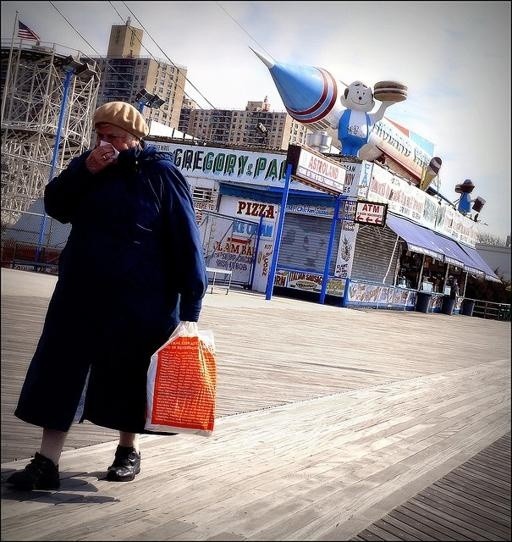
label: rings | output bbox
[102,154,107,161]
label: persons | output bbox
[0,99,210,491]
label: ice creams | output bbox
[422,157,442,191]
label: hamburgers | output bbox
[296,281,317,290]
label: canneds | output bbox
[273,273,287,287]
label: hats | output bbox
[93,101,149,140]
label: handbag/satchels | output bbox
[144,318,218,437]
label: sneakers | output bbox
[106,446,142,482]
[7,452,61,490]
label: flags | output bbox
[17,21,42,42]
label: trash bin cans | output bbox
[462,299,474,316]
[442,295,455,315]
[416,292,432,313]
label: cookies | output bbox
[374,81,408,102]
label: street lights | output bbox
[34,53,98,264]
